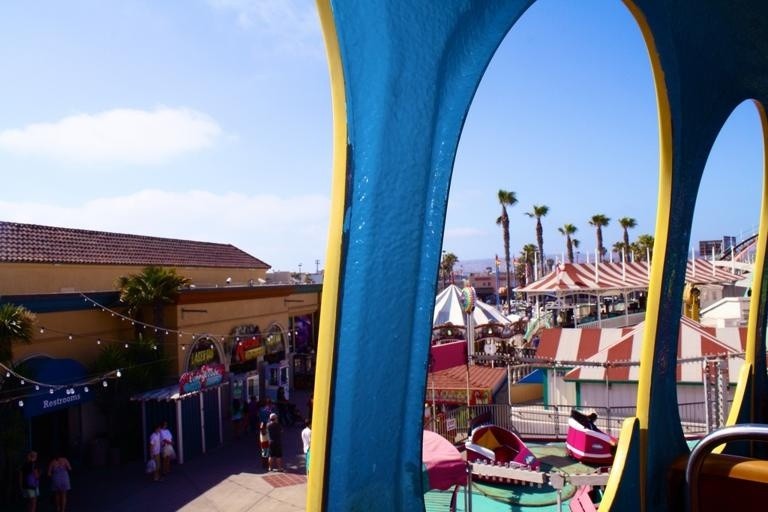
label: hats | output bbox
[269,412,278,419]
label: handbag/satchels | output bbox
[146,457,157,473]
[52,463,70,482]
[162,443,171,458]
[26,462,40,488]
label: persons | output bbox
[149,425,161,484]
[17,451,42,512]
[247,386,312,473]
[586,413,597,430]
[160,420,176,473]
[47,449,72,512]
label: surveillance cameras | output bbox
[226,277,232,284]
[258,278,266,284]
